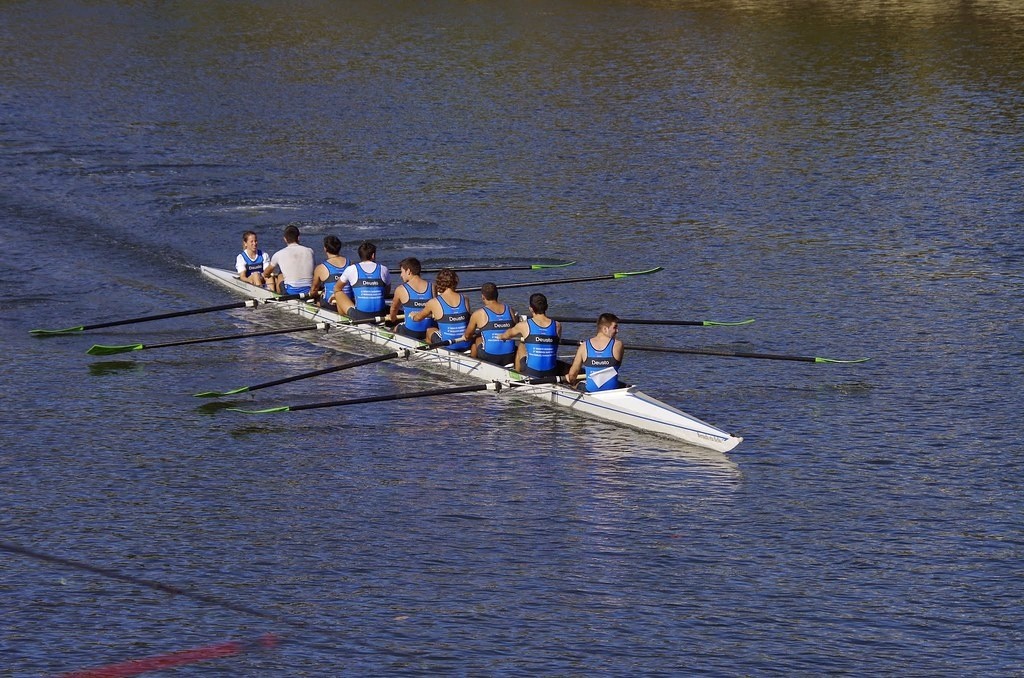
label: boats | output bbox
[198,265,743,453]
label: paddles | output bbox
[454,267,664,292]
[509,337,870,364]
[516,314,757,326]
[226,374,586,414]
[388,260,577,274]
[194,333,476,397]
[27,291,323,333]
[84,314,406,354]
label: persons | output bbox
[235,231,275,292]
[263,225,315,296]
[311,237,355,312]
[497,293,562,378]
[565,312,624,393]
[410,268,473,351]
[462,283,518,366]
[386,257,438,339]
[328,243,391,320]
